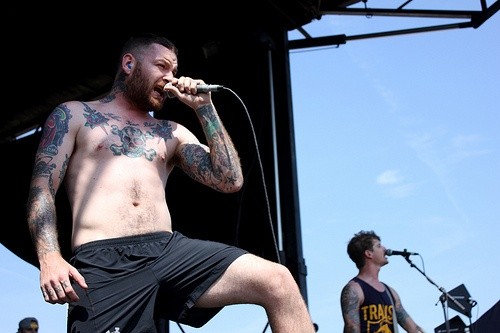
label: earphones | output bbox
[128,64,132,69]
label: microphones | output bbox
[387,249,419,256]
[164,82,223,98]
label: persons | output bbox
[27,31,317,333]
[340,231,424,333]
[17,316,39,333]
